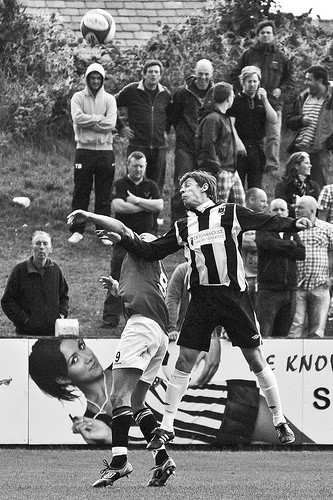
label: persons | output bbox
[163,151,333,338]
[287,196,333,338]
[258,198,306,339]
[96,171,313,444]
[114,60,173,196]
[283,65,333,202]
[168,59,214,228]
[99,151,164,330]
[226,21,293,180]
[28,336,316,444]
[194,81,246,207]
[67,209,176,487]
[239,188,269,327]
[227,66,277,191]
[67,63,117,245]
[0,231,69,337]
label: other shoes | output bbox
[68,232,82,243]
[101,237,113,245]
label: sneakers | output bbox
[146,456,177,488]
[275,422,295,445]
[146,427,175,449]
[91,464,133,487]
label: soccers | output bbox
[79,8,115,48]
